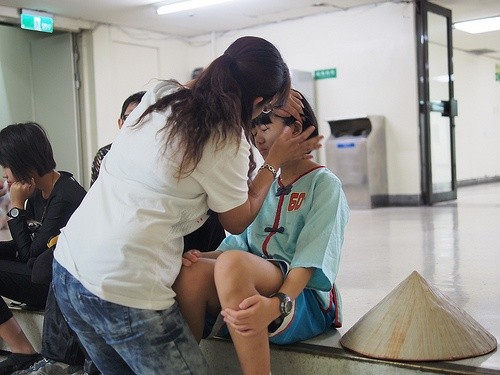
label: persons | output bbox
[52,36,325,375]
[172,89,348,375]
[0,91,147,375]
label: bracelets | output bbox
[259,164,278,180]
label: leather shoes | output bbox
[0,353,42,375]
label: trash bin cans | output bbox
[326,116,390,208]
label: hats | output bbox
[338,271,497,362]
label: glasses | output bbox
[262,102,273,113]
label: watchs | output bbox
[5,207,28,216]
[270,291,294,317]
